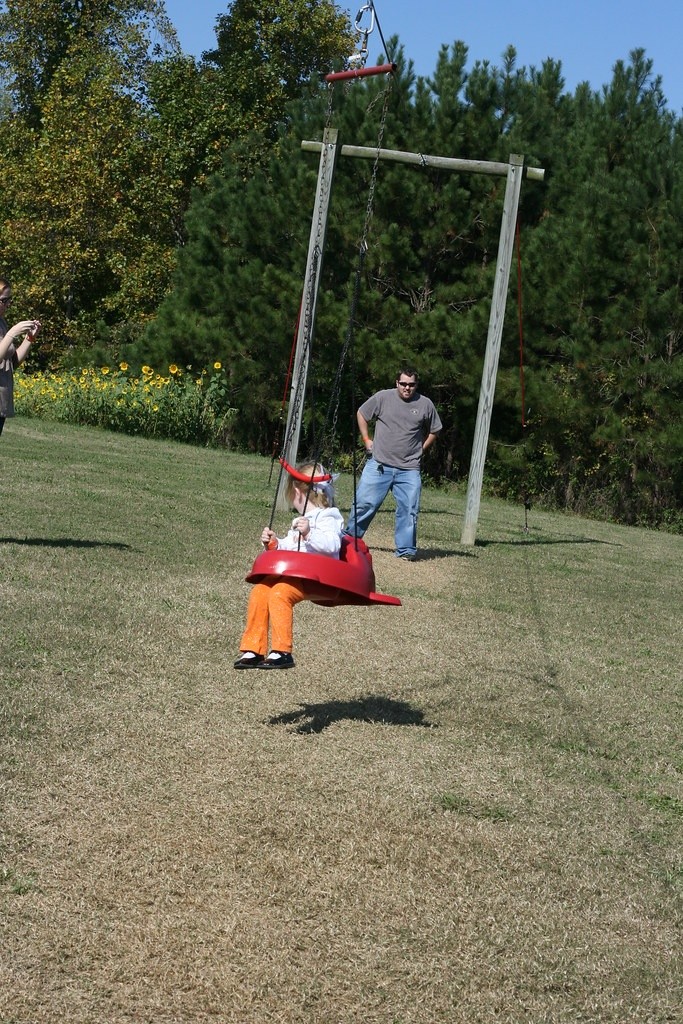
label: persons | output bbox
[344,367,444,561]
[233,461,343,670]
[0,278,42,437]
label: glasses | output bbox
[398,382,417,387]
[0,296,12,303]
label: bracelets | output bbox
[26,334,35,342]
[363,437,370,442]
[8,333,19,339]
[267,540,278,550]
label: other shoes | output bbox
[401,554,415,561]
[233,651,264,669]
[256,650,295,669]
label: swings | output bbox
[242,0,401,604]
